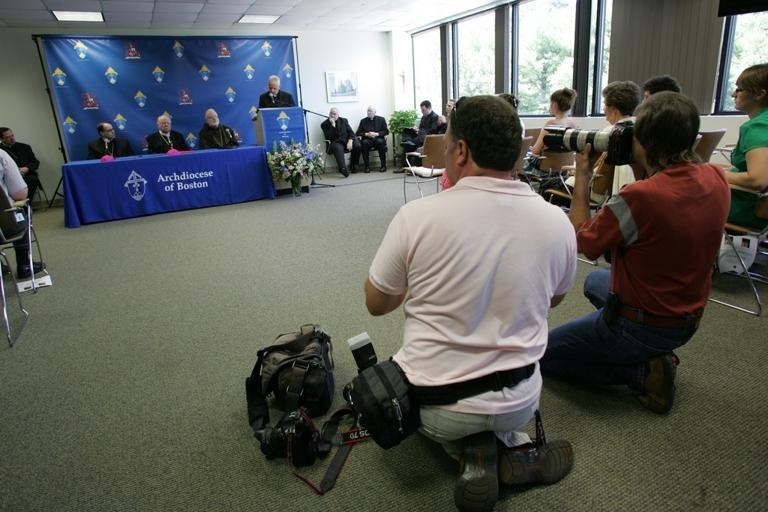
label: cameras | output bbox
[544,119,632,165]
[254,406,330,468]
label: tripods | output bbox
[297,110,336,190]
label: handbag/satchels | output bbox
[246,324,334,430]
[343,359,420,450]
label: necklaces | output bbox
[212,128,224,147]
[105,144,113,156]
[160,132,173,146]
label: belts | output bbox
[620,308,689,328]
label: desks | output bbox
[63,145,277,227]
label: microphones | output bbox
[225,128,233,139]
[269,93,276,103]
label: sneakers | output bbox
[455,431,573,512]
[639,355,677,414]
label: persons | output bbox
[539,90,732,415]
[494,93,525,142]
[351,95,578,512]
[394,99,456,173]
[88,122,136,160]
[198,109,239,150]
[0,127,40,215]
[723,65,768,233]
[0,147,47,279]
[528,75,681,208]
[145,115,191,154]
[259,75,296,109]
[320,107,361,177]
[355,106,389,173]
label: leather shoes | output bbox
[17,262,46,278]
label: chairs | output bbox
[405,129,768,317]
[323,136,356,175]
[0,252,28,347]
[0,185,48,294]
[356,136,387,171]
